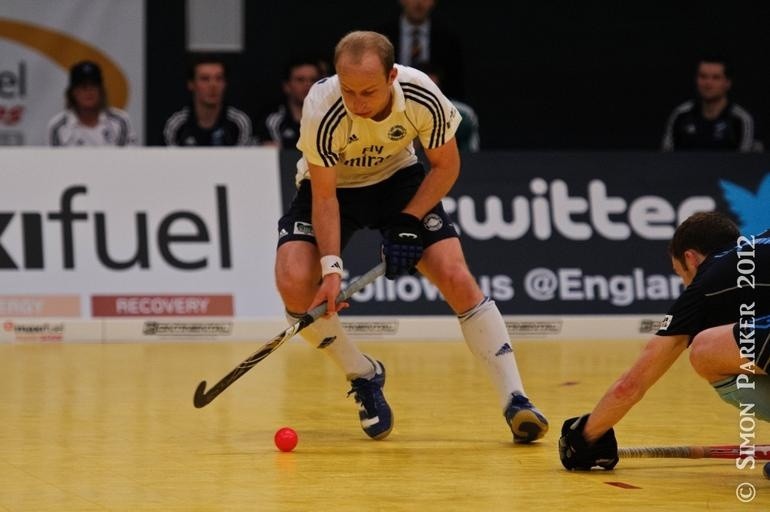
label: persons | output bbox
[659,56,757,152]
[382,0,460,87]
[262,59,324,149]
[558,210,770,481]
[274,29,551,445]
[161,58,257,146]
[43,58,138,146]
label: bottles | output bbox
[71,61,101,87]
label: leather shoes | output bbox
[559,414,619,471]
[381,214,424,280]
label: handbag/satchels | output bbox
[319,254,344,279]
[392,212,421,233]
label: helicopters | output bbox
[275,428,297,451]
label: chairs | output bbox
[345,353,393,440]
[505,392,548,444]
